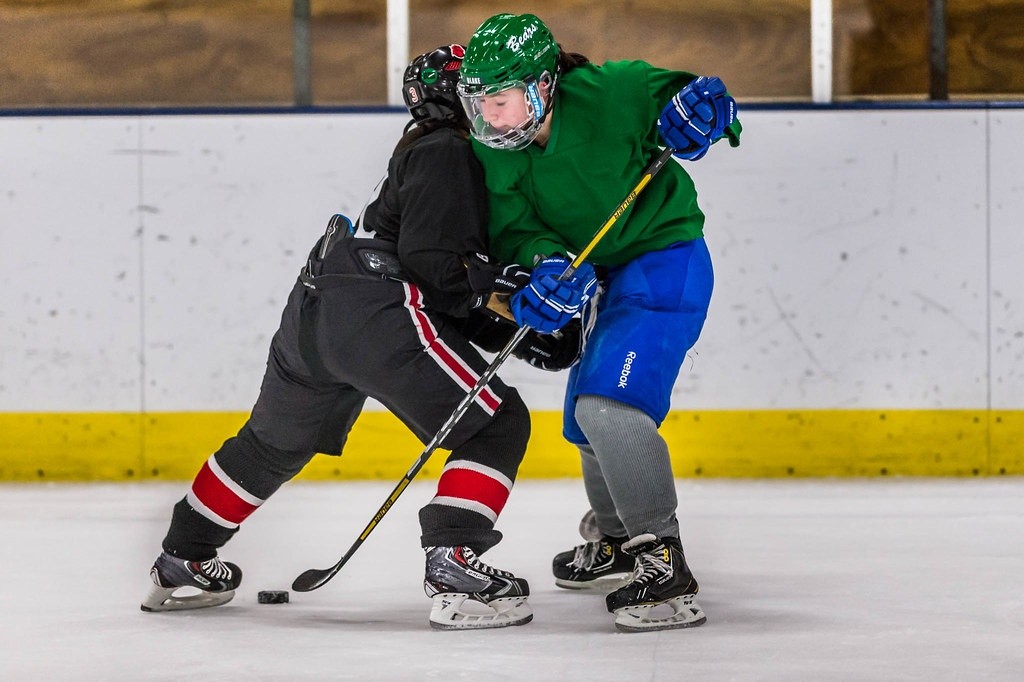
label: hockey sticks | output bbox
[292,147,672,593]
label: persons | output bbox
[455,13,743,630]
[140,43,586,630]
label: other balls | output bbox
[257,590,289,604]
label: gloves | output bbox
[510,257,597,334]
[655,75,737,161]
[512,318,581,372]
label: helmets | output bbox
[455,13,560,151]
[402,43,471,133]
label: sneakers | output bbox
[606,533,706,633]
[552,509,635,590]
[140,550,242,612]
[423,546,533,630]
